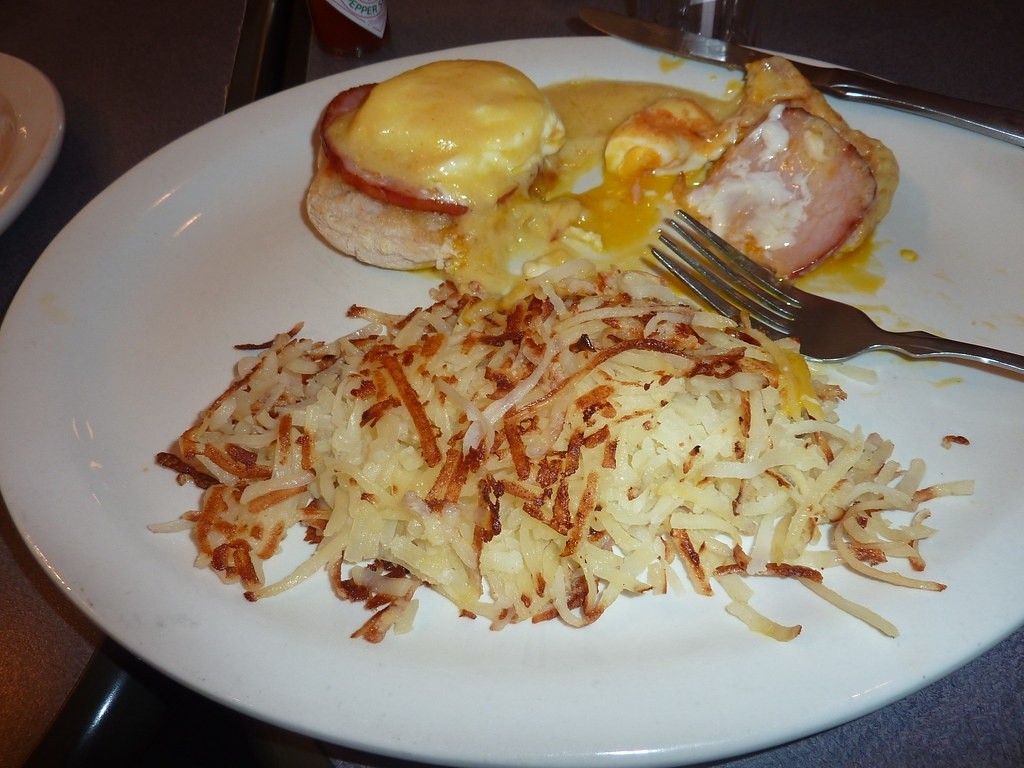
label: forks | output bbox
[651,209,1024,378]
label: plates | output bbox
[0,34,1024,768]
[1,54,65,237]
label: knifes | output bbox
[577,7,1024,153]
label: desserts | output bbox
[305,59,566,271]
